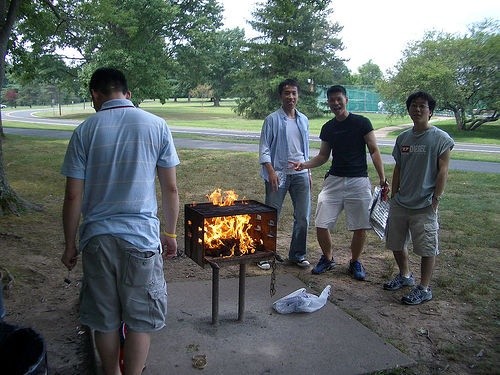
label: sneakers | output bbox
[288,257,310,266]
[349,259,365,281]
[256,260,271,270]
[401,284,433,305]
[311,255,336,275]
[382,272,414,291]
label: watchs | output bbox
[433,195,442,201]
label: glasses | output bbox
[410,103,429,108]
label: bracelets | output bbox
[164,231,177,238]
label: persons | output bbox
[288,85,390,279]
[60,66,181,375]
[257,79,313,269]
[385,91,454,305]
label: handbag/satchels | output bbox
[272,285,331,314]
